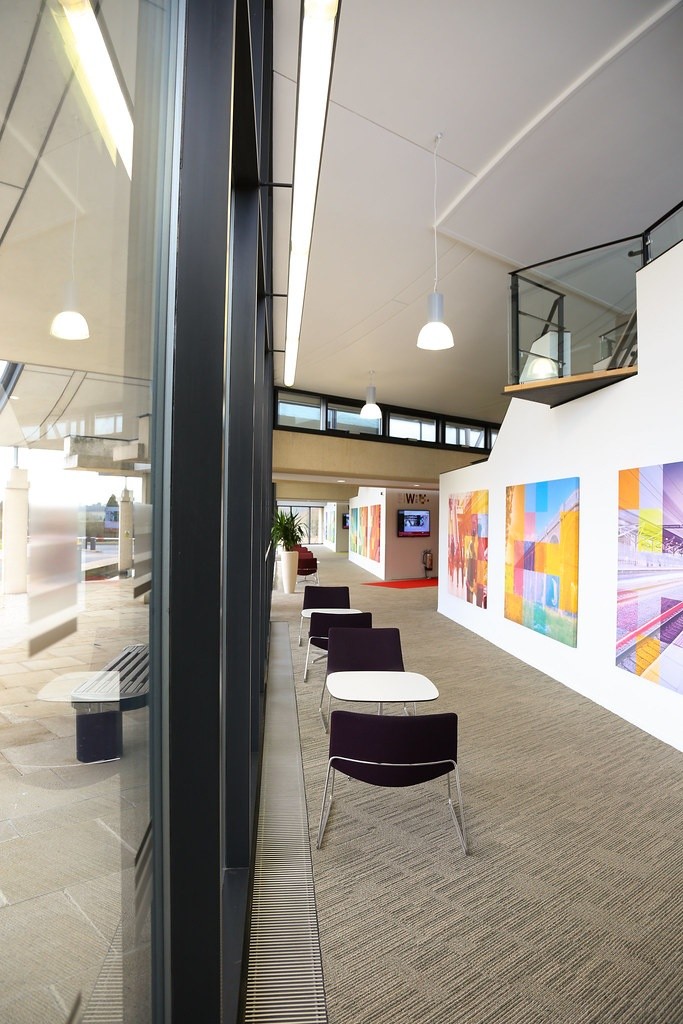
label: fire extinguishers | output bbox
[422,550,433,571]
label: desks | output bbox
[300,608,364,664]
[325,671,440,783]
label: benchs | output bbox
[68,634,150,762]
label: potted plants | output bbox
[269,507,311,594]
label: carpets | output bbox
[362,577,438,591]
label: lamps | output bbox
[527,357,558,378]
[415,129,455,351]
[361,370,384,420]
[51,127,92,341]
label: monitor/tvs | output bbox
[398,509,431,537]
[342,513,349,529]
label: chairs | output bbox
[313,706,470,856]
[318,626,417,734]
[304,611,373,683]
[292,543,319,586]
[298,585,351,646]
